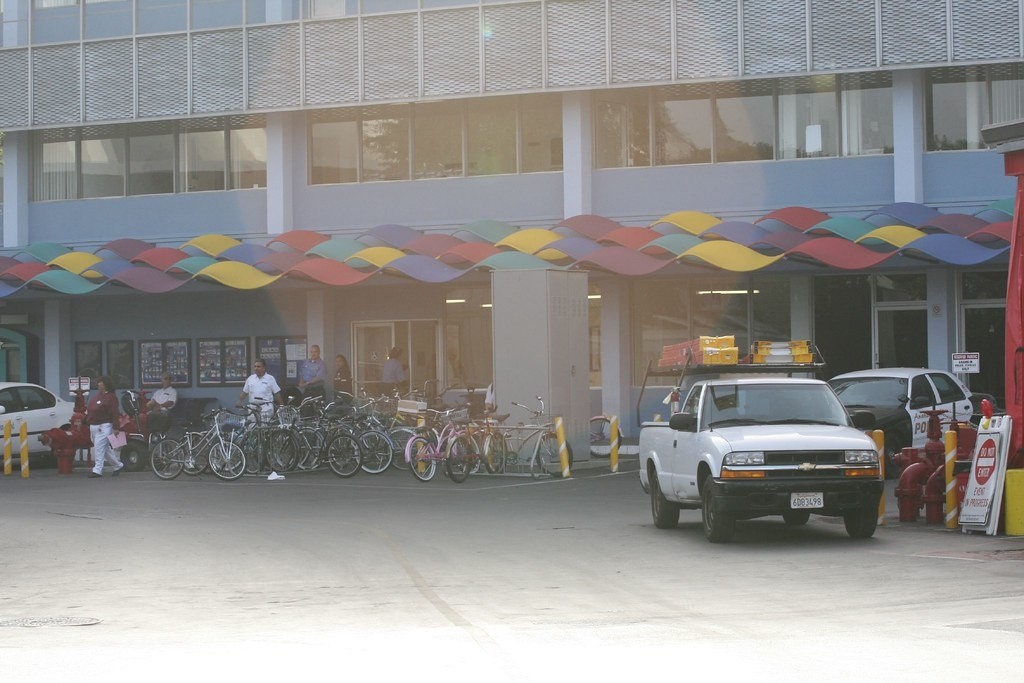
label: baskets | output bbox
[532,412,563,431]
[212,395,469,432]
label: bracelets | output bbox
[306,382,310,385]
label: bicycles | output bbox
[588,415,624,458]
[147,379,573,483]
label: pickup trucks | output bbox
[637,377,883,544]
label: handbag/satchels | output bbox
[108,431,128,448]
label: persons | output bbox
[235,358,285,429]
[83,375,124,479]
[484,380,495,413]
[299,344,327,400]
[145,372,178,433]
[334,355,352,403]
[381,346,407,396]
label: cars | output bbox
[824,366,997,482]
[0,380,89,460]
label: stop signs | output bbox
[974,438,997,484]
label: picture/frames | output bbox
[72,337,251,388]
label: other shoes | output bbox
[113,467,124,476]
[89,473,101,477]
